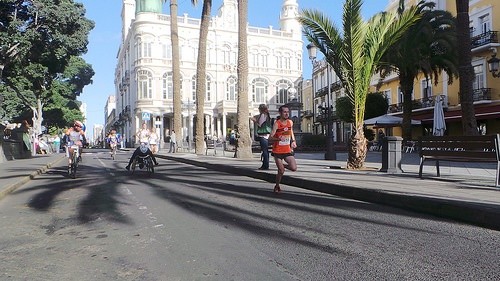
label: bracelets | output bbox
[292,140,296,142]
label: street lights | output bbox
[306,36,337,160]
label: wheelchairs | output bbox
[127,152,156,175]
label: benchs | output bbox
[417,133,500,187]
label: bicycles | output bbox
[110,142,119,160]
[64,140,85,178]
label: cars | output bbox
[204,135,223,148]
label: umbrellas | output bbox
[363,115,421,126]
[433,93,446,136]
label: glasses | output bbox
[75,124,79,127]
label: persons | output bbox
[168,130,176,153]
[137,123,150,147]
[378,130,385,150]
[252,104,271,170]
[149,127,159,154]
[63,121,89,171]
[106,130,119,157]
[268,105,297,192]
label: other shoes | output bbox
[78,157,82,161]
[274,186,281,192]
[259,165,268,169]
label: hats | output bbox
[76,121,83,126]
[258,104,266,109]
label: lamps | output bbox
[487,55,500,78]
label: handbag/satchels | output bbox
[256,112,271,134]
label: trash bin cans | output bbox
[380,136,403,172]
[206,139,216,148]
